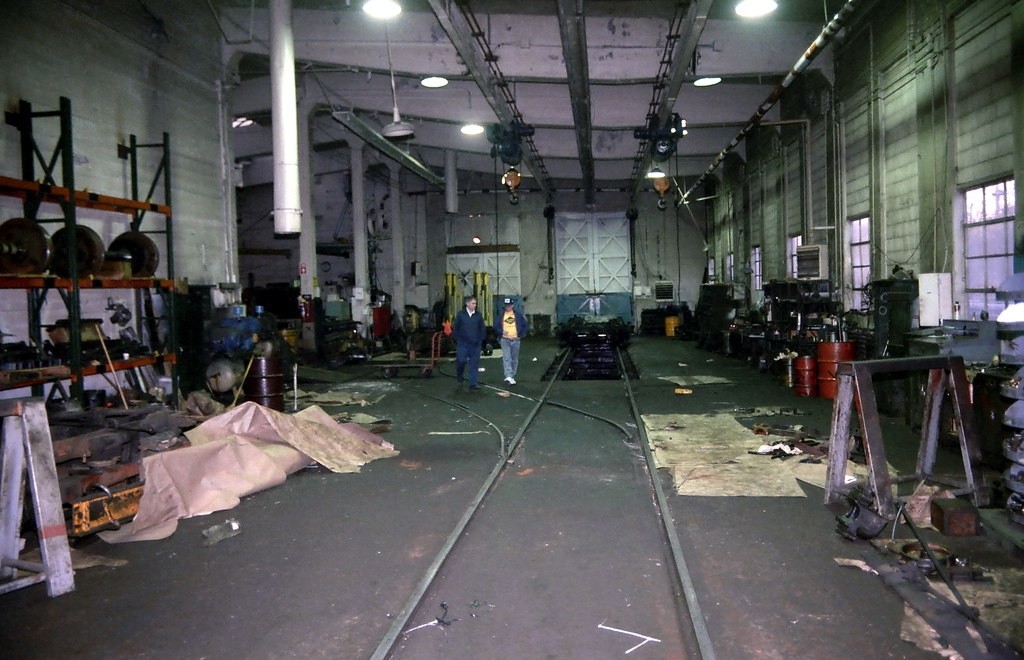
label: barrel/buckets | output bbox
[816,340,854,400]
[793,357,818,398]
[664,316,680,337]
[241,355,287,414]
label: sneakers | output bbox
[455,385,463,392]
[503,377,512,388]
[469,385,481,391]
[510,377,517,385]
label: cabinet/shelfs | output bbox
[0,175,178,412]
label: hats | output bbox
[503,297,515,305]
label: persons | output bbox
[451,295,486,390]
[492,298,530,384]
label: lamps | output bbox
[358,0,783,210]
